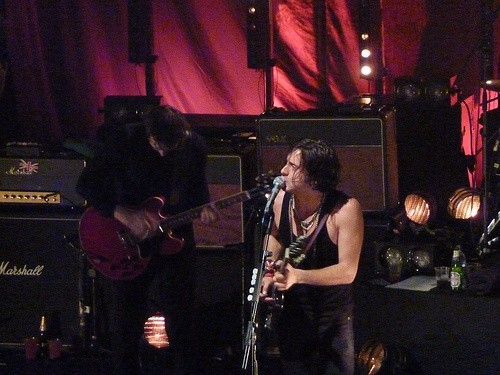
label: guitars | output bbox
[264,239,307,332]
[78,169,279,280]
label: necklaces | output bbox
[291,206,321,230]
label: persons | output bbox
[259,138,364,375]
[80,107,217,348]
[0,60,18,154]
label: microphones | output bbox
[263,177,285,216]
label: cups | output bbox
[434,266,450,289]
[24,339,37,360]
[49,338,61,359]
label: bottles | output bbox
[37,312,49,358]
[450,248,463,291]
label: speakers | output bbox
[0,212,90,351]
[392,100,461,203]
[128,0,154,65]
[358,0,384,81]
[246,0,271,69]
[186,153,245,250]
[256,114,390,216]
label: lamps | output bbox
[403,187,482,225]
